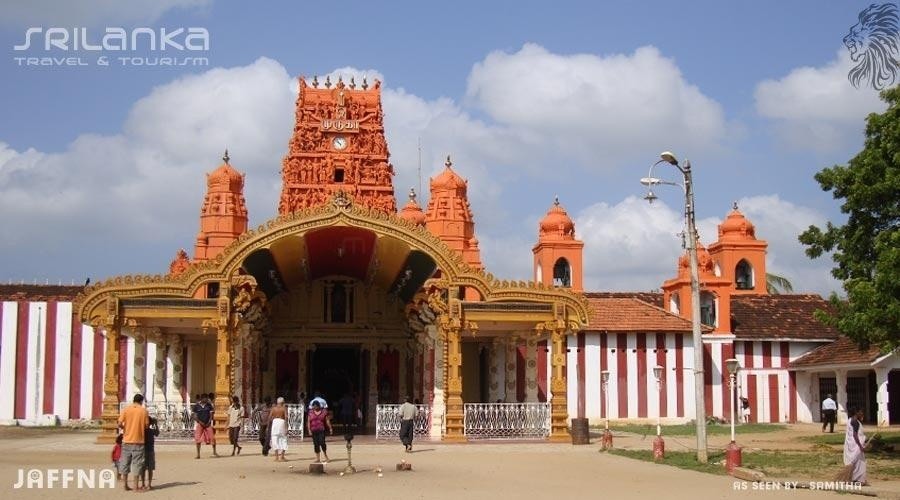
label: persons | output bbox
[844,404,873,487]
[279,99,398,217]
[110,395,160,491]
[190,392,423,467]
[740,395,751,424]
[821,393,837,433]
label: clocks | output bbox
[333,137,347,150]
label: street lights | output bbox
[599,369,613,449]
[641,151,710,466]
[721,358,743,472]
[651,362,666,462]
